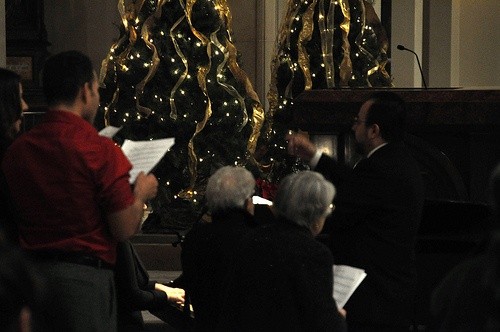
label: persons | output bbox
[0,67,29,332]
[283,89,423,331]
[181,163,259,332]
[0,49,159,332]
[114,238,185,332]
[251,169,349,332]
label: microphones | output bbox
[397,45,427,88]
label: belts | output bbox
[22,248,111,270]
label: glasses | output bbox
[352,115,367,125]
